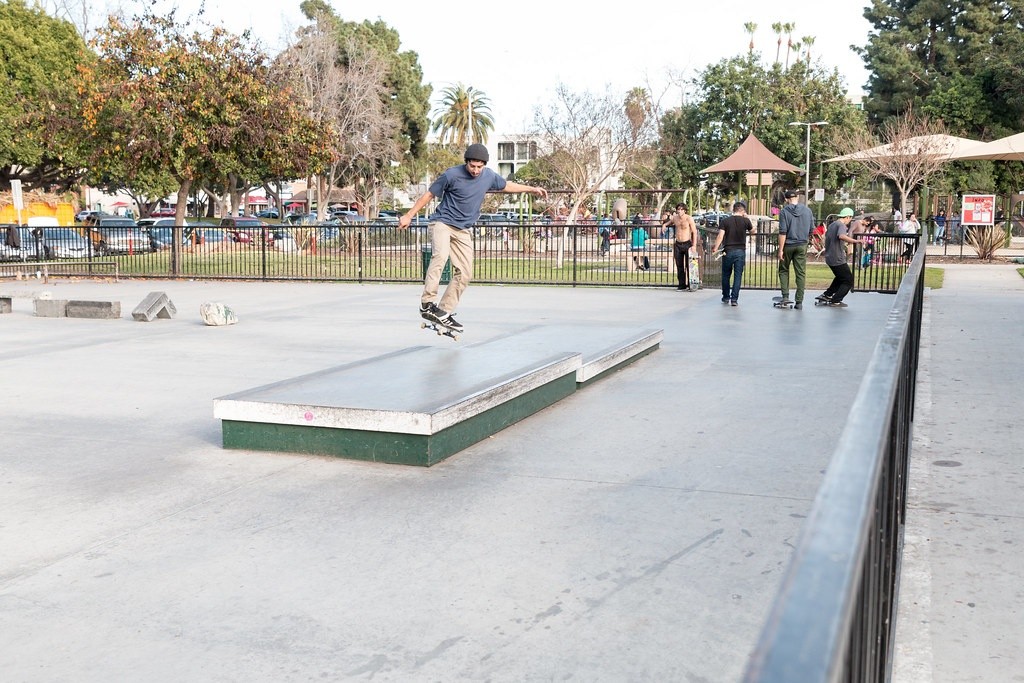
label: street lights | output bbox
[789,121,829,207]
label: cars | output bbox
[31,226,94,260]
[75,211,432,251]
[0,223,47,262]
[475,212,737,235]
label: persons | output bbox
[777,191,815,310]
[662,203,698,290]
[811,206,1005,264]
[712,202,755,306]
[398,144,548,331]
[822,207,864,305]
[504,203,707,270]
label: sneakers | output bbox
[443,314,464,332]
[419,303,448,321]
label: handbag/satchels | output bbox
[897,229,906,233]
[863,241,869,251]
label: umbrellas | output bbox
[287,190,359,213]
[824,131,1024,164]
[698,132,805,214]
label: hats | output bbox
[837,207,854,217]
[785,191,797,199]
[464,143,488,163]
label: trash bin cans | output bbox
[422,248,450,286]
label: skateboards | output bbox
[814,295,848,308]
[689,246,702,292]
[421,311,464,341]
[711,245,726,262]
[772,296,794,310]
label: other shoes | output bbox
[794,302,802,310]
[830,301,847,308]
[905,259,910,263]
[823,292,832,299]
[731,299,738,306]
[899,255,903,263]
[676,287,689,291]
[721,297,729,303]
